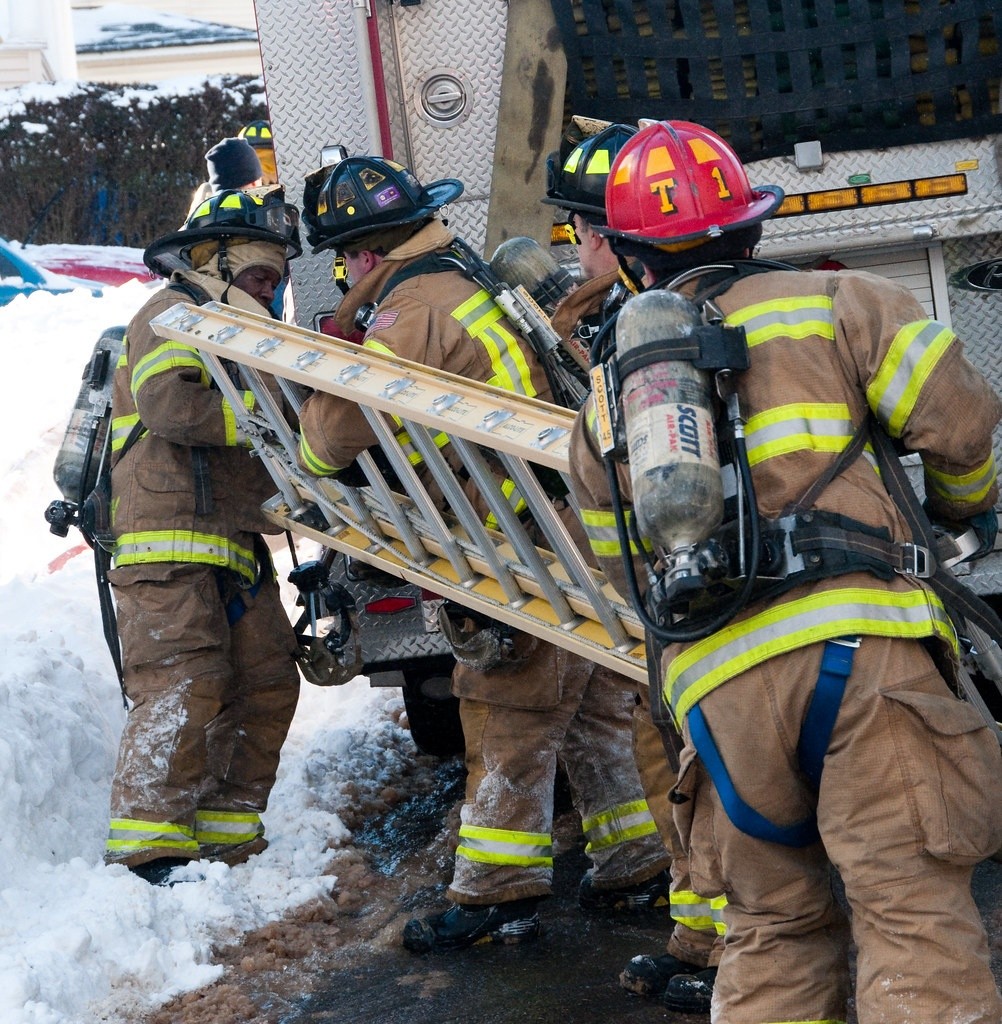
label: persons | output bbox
[295,125,729,998]
[104,119,303,890]
[567,118,1002,1024]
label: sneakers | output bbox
[578,870,671,915]
[621,953,701,996]
[663,967,717,1012]
[402,904,542,954]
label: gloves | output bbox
[921,497,999,563]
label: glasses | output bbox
[246,203,299,239]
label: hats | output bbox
[204,137,263,193]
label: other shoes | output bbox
[128,856,207,890]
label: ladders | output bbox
[146,299,663,686]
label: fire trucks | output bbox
[256,0,1002,761]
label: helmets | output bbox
[238,120,273,148]
[301,156,464,254]
[589,120,785,251]
[143,189,303,280]
[540,124,639,214]
